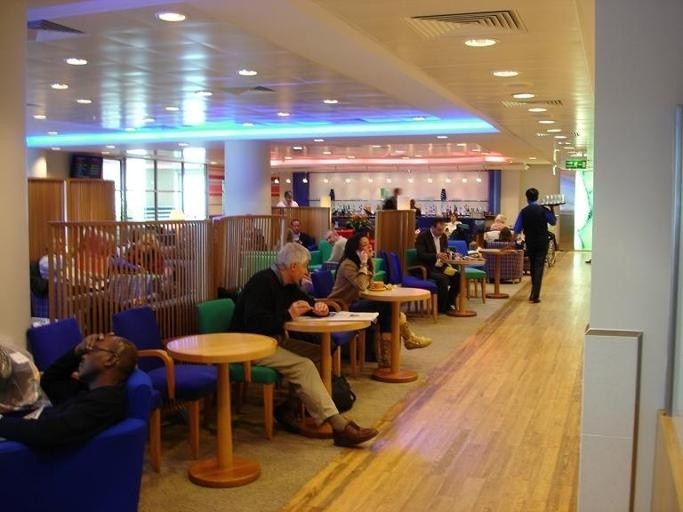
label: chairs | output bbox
[26,317,163,470]
[390,251,440,323]
[405,248,438,283]
[447,240,484,271]
[447,247,486,304]
[380,250,419,284]
[196,298,305,440]
[112,305,218,461]
[371,258,386,281]
[310,270,382,372]
[241,240,337,282]
[281,301,357,379]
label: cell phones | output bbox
[355,249,361,260]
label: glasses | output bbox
[85,342,122,359]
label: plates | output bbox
[370,288,386,291]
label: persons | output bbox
[239,214,266,252]
[39,230,187,303]
[445,212,463,237]
[277,191,300,207]
[472,230,489,247]
[468,241,485,259]
[0,333,138,461]
[416,220,460,314]
[411,200,420,216]
[323,229,348,263]
[329,234,432,364]
[496,227,513,243]
[490,215,507,230]
[513,188,556,304]
[383,188,402,210]
[287,220,317,251]
[230,243,379,446]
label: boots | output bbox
[379,333,392,368]
[399,312,433,350]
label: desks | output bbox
[479,248,517,298]
[441,260,486,317]
[166,332,279,488]
[359,287,432,382]
[282,312,372,439]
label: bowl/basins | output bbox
[374,281,383,288]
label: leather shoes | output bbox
[273,398,303,435]
[438,304,454,314]
[332,419,379,448]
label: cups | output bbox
[539,194,564,204]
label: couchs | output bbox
[0,368,153,512]
[486,241,524,284]
[66,273,153,313]
[31,258,136,316]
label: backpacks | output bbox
[332,375,356,413]
